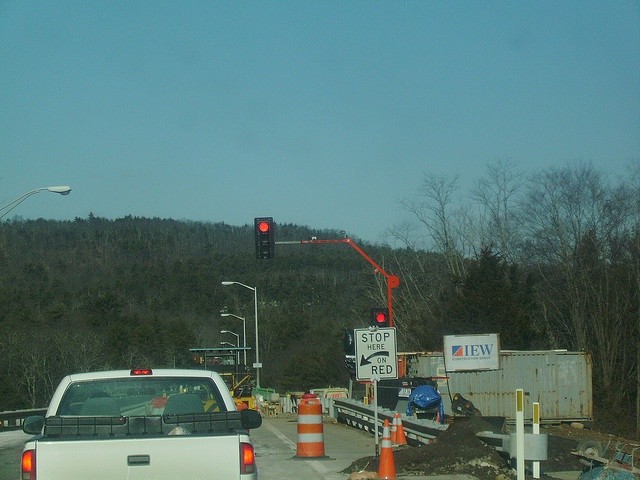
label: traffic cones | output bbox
[391,414,396,442]
[396,414,407,444]
[297,394,325,457]
[379,420,396,479]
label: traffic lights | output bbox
[254,217,274,259]
[371,308,389,328]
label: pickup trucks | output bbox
[20,369,261,480]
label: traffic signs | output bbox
[354,326,399,381]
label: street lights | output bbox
[222,282,262,395]
[220,341,236,354]
[221,313,246,369]
[0,186,70,217]
[220,330,240,366]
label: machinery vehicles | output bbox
[201,373,257,412]
